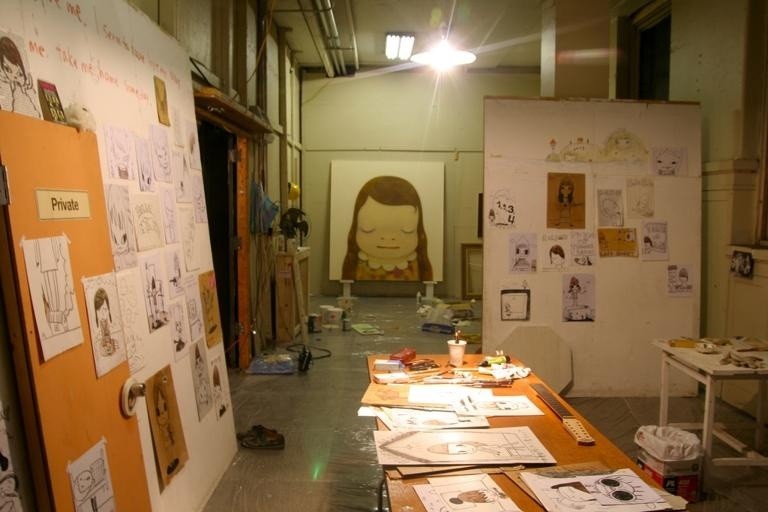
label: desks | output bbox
[366,353,670,512]
[653,338,768,480]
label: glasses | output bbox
[599,479,636,503]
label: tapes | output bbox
[122,376,140,418]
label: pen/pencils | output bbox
[455,331,459,344]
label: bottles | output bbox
[278,230,297,254]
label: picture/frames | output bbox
[461,243,484,300]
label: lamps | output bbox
[385,32,415,60]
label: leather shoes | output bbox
[237,424,285,450]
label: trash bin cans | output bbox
[634,425,702,505]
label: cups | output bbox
[448,340,466,366]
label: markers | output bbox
[482,355,510,367]
